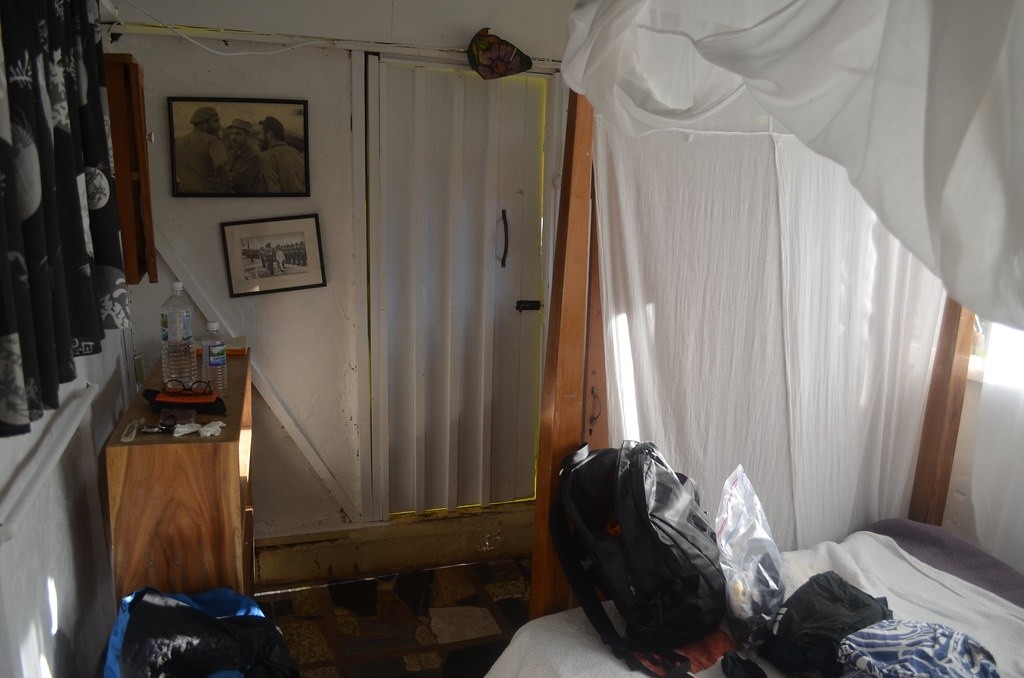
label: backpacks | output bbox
[549,438,723,678]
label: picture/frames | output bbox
[218,212,330,302]
[165,93,315,200]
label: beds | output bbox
[460,515,1024,678]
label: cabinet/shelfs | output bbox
[100,336,256,605]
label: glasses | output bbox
[162,379,212,396]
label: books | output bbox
[195,336,250,356]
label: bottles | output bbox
[161,281,198,389]
[201,321,228,392]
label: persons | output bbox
[226,117,268,194]
[256,116,306,195]
[174,106,231,193]
[241,240,307,277]
